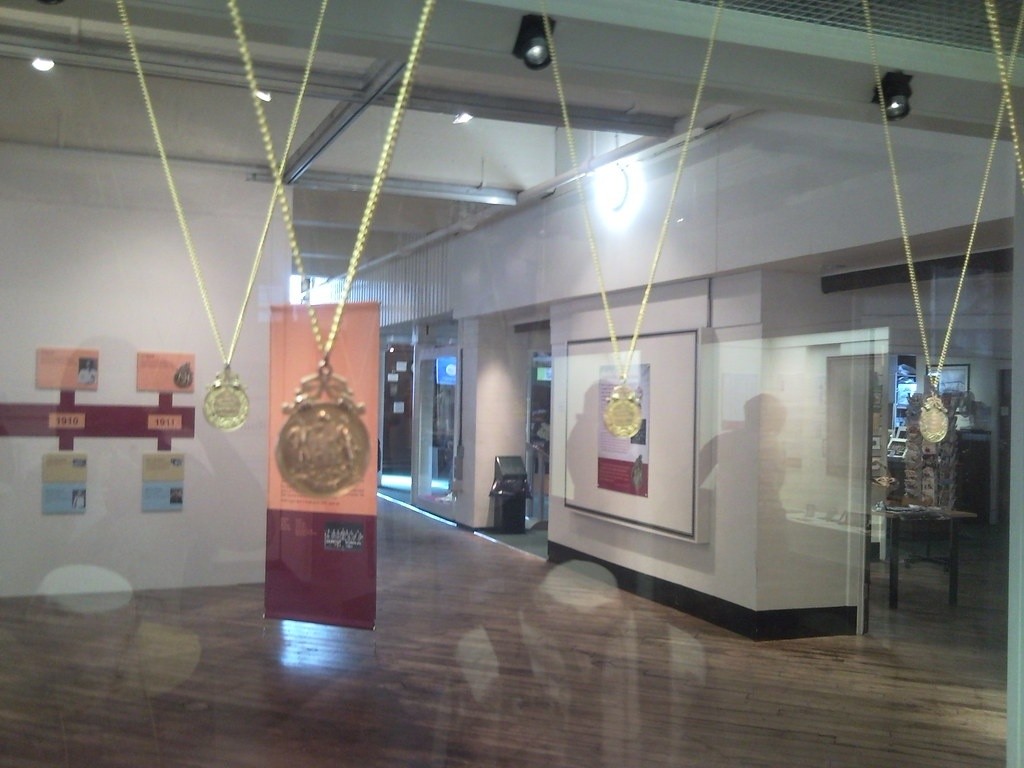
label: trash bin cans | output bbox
[490,455,527,534]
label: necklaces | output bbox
[114,0,441,501]
[536,1,725,439]
[859,0,1024,443]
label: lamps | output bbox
[870,71,914,122]
[511,13,557,72]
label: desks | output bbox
[871,506,978,610]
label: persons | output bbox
[73,490,85,509]
[79,359,97,384]
[697,394,787,559]
